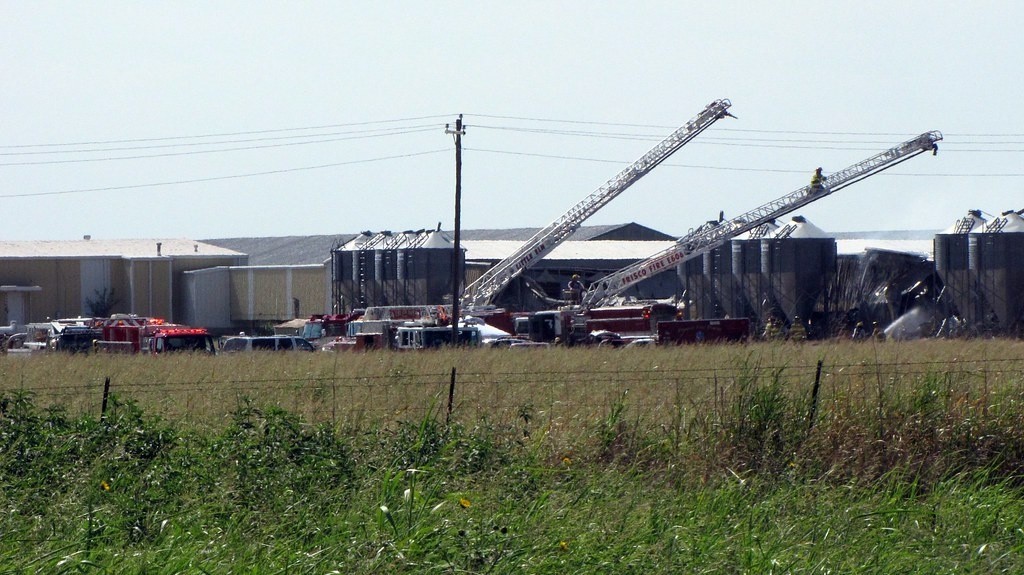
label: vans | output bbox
[221,334,316,352]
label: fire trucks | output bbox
[0,314,216,355]
[300,97,740,355]
[513,129,943,347]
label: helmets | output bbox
[768,316,775,321]
[572,274,580,280]
[872,321,877,326]
[816,166,823,170]
[793,316,800,322]
[857,322,863,327]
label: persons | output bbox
[807,167,827,194]
[852,321,886,342]
[765,316,808,342]
[567,274,588,305]
